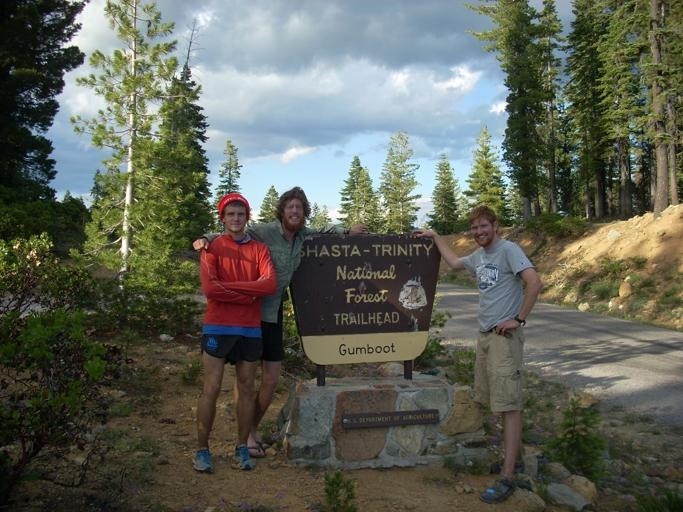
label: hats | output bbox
[216,192,251,222]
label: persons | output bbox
[192,188,365,459]
[411,205,544,505]
[192,192,278,473]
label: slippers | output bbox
[247,440,275,458]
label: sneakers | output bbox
[487,450,526,475]
[480,478,517,504]
[233,445,252,471]
[190,448,215,473]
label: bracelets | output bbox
[513,314,526,326]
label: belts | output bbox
[493,325,513,339]
